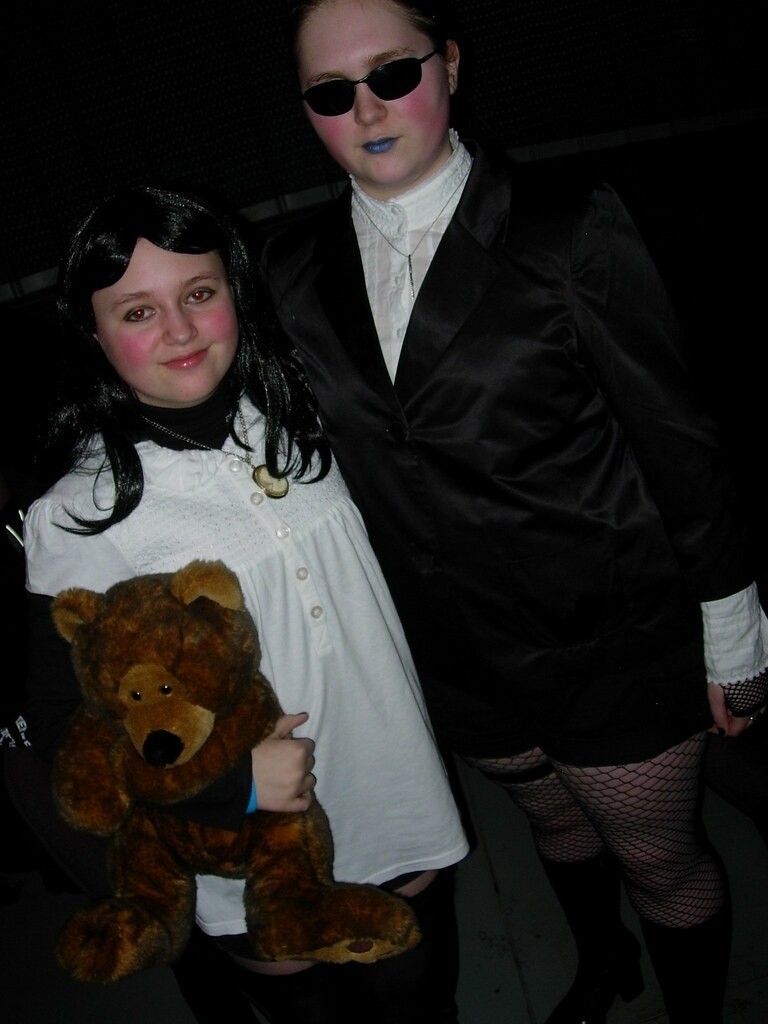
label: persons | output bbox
[262,1,768,1022]
[25,180,469,1024]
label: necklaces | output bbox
[142,386,291,499]
[354,163,473,297]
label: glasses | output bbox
[295,43,447,116]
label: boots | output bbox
[638,896,733,1024]
[539,852,645,1024]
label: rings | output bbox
[749,713,763,721]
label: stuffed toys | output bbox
[53,561,424,984]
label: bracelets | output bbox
[245,780,256,814]
